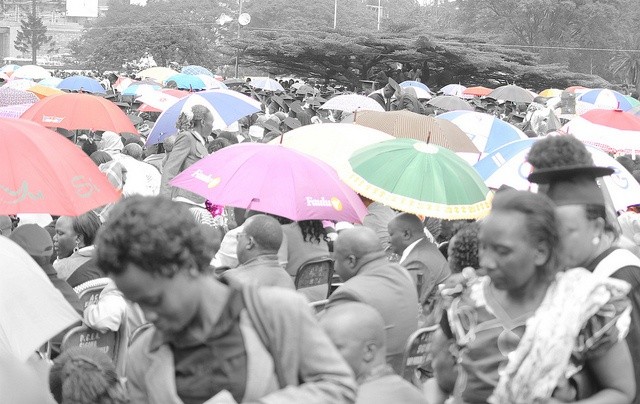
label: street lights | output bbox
[235,13,251,78]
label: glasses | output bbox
[236,232,248,240]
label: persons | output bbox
[210,209,288,272]
[161,104,216,196]
[613,152,639,180]
[81,130,175,194]
[368,77,401,109]
[115,95,159,135]
[389,213,452,315]
[373,60,431,83]
[276,77,356,99]
[92,192,357,404]
[522,131,638,370]
[208,123,300,149]
[360,79,385,109]
[428,226,480,358]
[54,126,74,141]
[429,189,637,403]
[327,225,422,375]
[278,216,329,280]
[96,71,116,97]
[7,221,82,314]
[356,192,395,252]
[49,67,98,78]
[216,213,296,292]
[314,300,426,404]
[485,100,570,137]
[121,64,144,80]
[48,343,125,404]
[53,210,107,288]
[82,281,149,376]
[391,86,425,115]
[242,90,329,123]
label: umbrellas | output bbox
[0,112,119,215]
[169,139,368,230]
[0,234,84,391]
[166,73,224,91]
[463,85,493,96]
[251,75,283,92]
[0,62,60,88]
[341,105,481,155]
[137,65,173,83]
[0,85,38,116]
[486,81,533,105]
[278,122,392,162]
[539,81,583,100]
[27,84,58,99]
[473,125,638,210]
[181,64,213,77]
[441,80,471,100]
[124,82,160,96]
[319,93,385,114]
[222,77,244,85]
[397,79,431,99]
[18,88,139,135]
[436,105,526,164]
[141,83,264,152]
[426,93,473,114]
[577,86,631,111]
[338,130,492,225]
[559,101,638,155]
[135,88,189,112]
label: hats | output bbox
[9,223,54,256]
